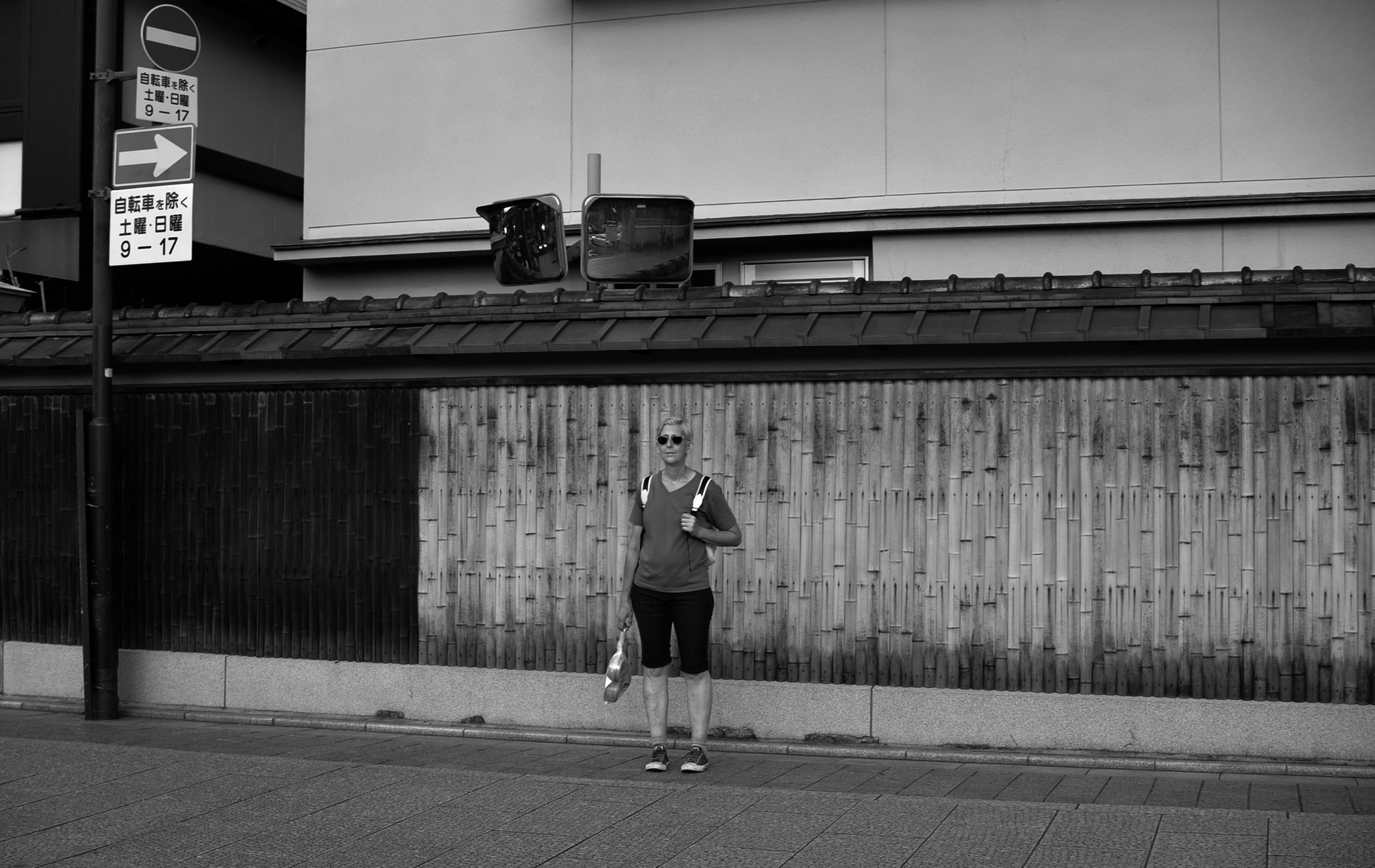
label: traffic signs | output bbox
[111,124,194,265]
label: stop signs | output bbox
[138,4,202,71]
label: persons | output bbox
[615,416,742,772]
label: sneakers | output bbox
[645,743,670,770]
[681,745,709,772]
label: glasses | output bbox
[655,435,685,446]
